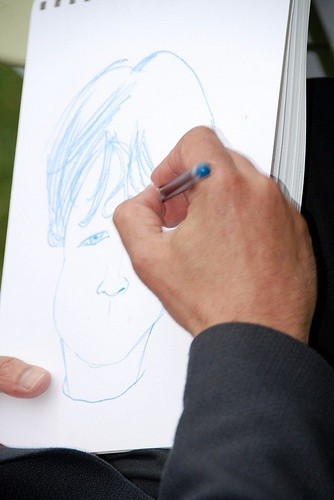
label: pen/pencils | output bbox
[156,163,210,203]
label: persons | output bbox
[0,123,334,500]
